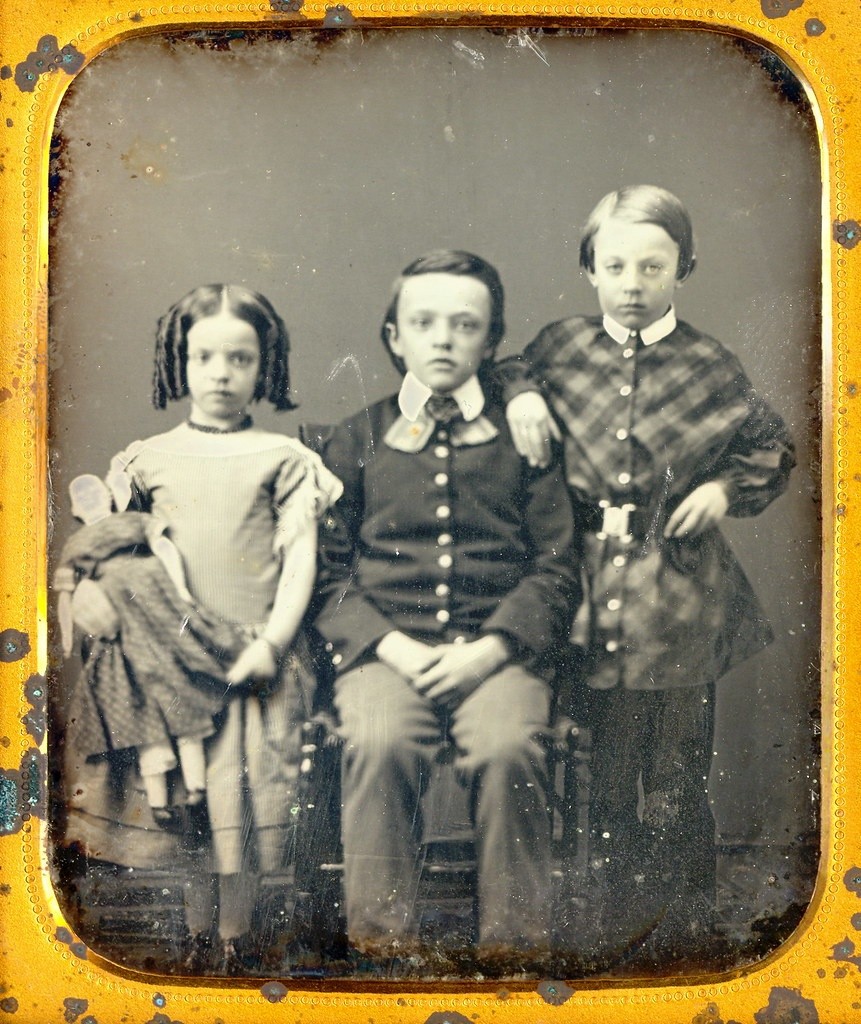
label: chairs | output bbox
[298,419,595,940]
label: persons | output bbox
[314,250,579,964]
[491,183,798,946]
[55,475,245,829]
[70,283,344,975]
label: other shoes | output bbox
[179,930,210,975]
[218,937,247,978]
